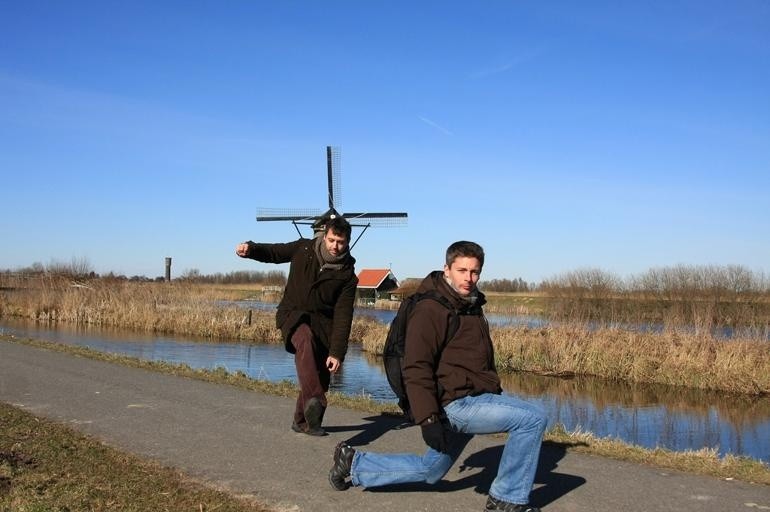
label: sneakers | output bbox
[303,396,324,431]
[291,420,326,436]
[328,441,356,492]
[483,494,541,512]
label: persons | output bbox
[329,241,547,511]
[237,217,359,436]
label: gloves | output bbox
[421,417,451,455]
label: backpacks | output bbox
[382,289,461,425]
